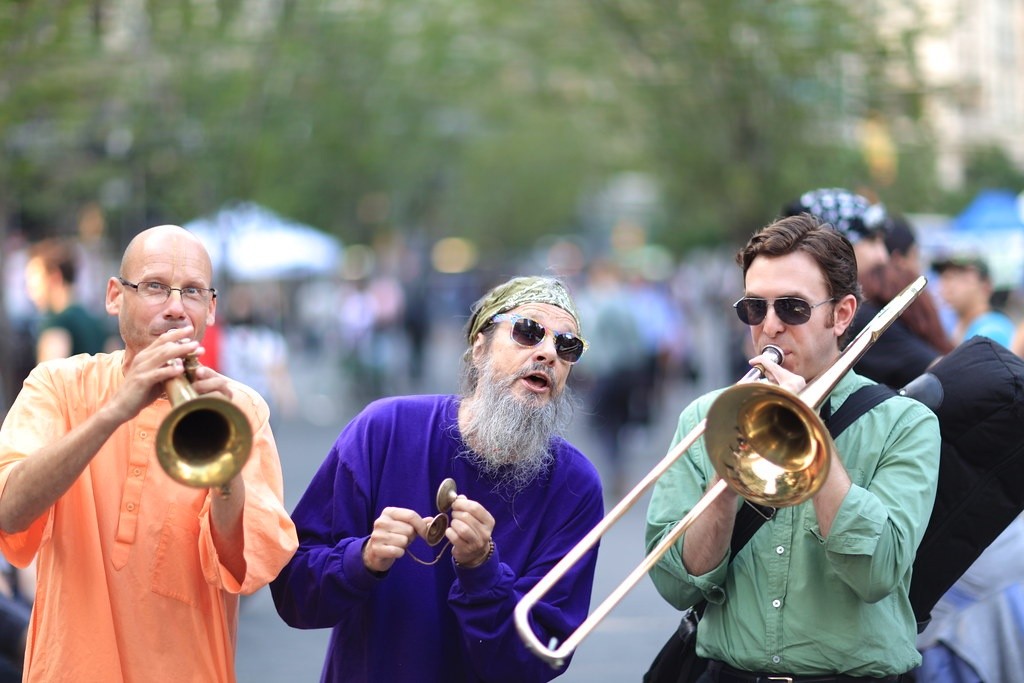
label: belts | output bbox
[710,661,836,683]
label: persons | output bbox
[269,274,604,683]
[0,223,299,683]
[0,236,755,504]
[644,188,1024,683]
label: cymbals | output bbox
[423,476,458,548]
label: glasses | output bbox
[489,314,591,365]
[119,277,217,310]
[733,296,834,325]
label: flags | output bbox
[184,204,340,280]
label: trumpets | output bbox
[152,326,254,489]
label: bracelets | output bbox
[456,537,494,569]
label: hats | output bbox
[932,247,989,273]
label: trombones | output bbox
[511,275,930,669]
